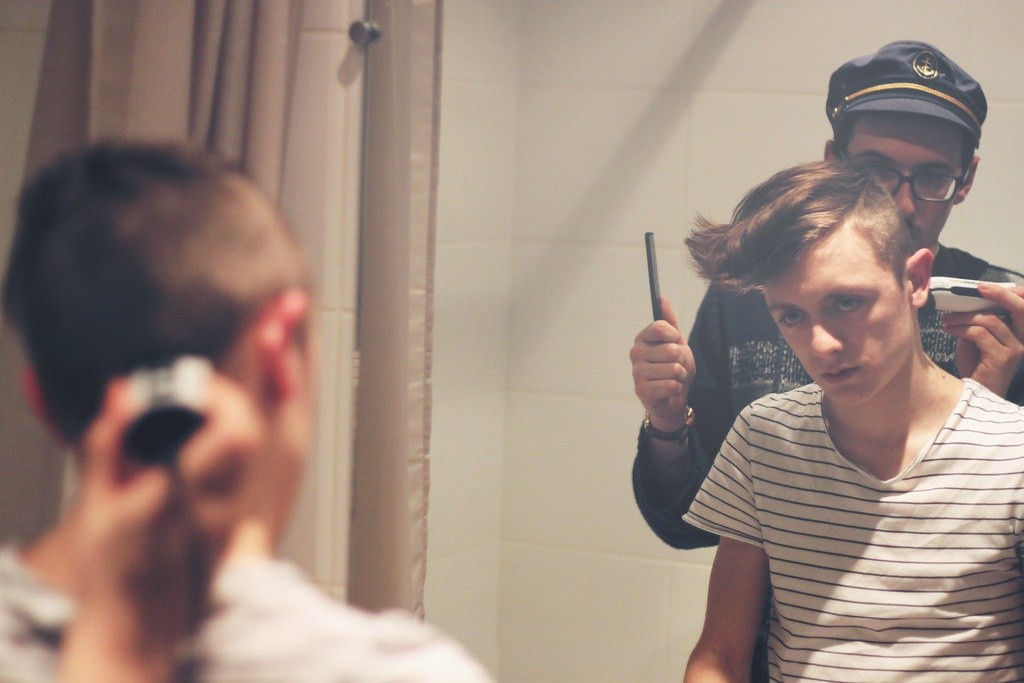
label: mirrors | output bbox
[347,0,1024,683]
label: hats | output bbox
[826,40,988,150]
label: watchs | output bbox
[644,405,694,446]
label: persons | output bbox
[54,371,268,682]
[0,137,490,683]
[682,161,1024,683]
[629,41,1024,550]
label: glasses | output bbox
[840,151,975,204]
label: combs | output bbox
[645,232,663,322]
